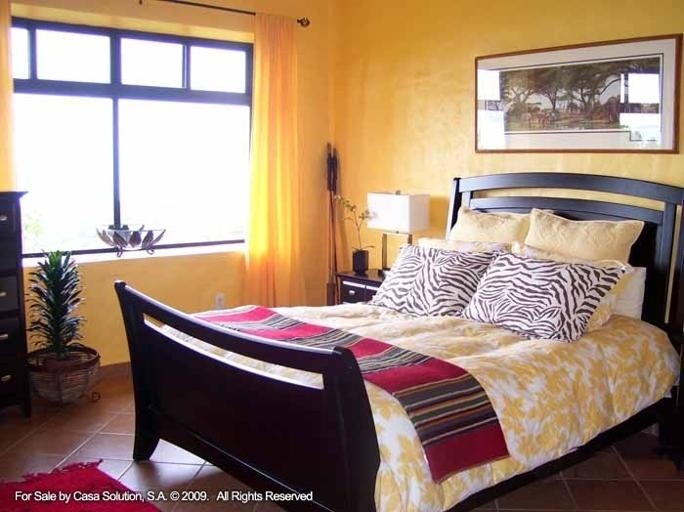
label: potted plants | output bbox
[339,198,375,272]
[24,251,102,408]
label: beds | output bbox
[114,172,684,512]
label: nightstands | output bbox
[336,269,384,304]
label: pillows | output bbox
[367,244,500,317]
[449,208,553,249]
[420,238,504,252]
[513,242,633,332]
[464,250,624,343]
[613,267,646,320]
[526,208,643,264]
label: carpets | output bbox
[0,460,162,512]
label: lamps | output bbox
[368,191,431,274]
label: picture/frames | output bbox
[475,34,682,155]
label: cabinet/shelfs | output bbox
[0,191,32,418]
